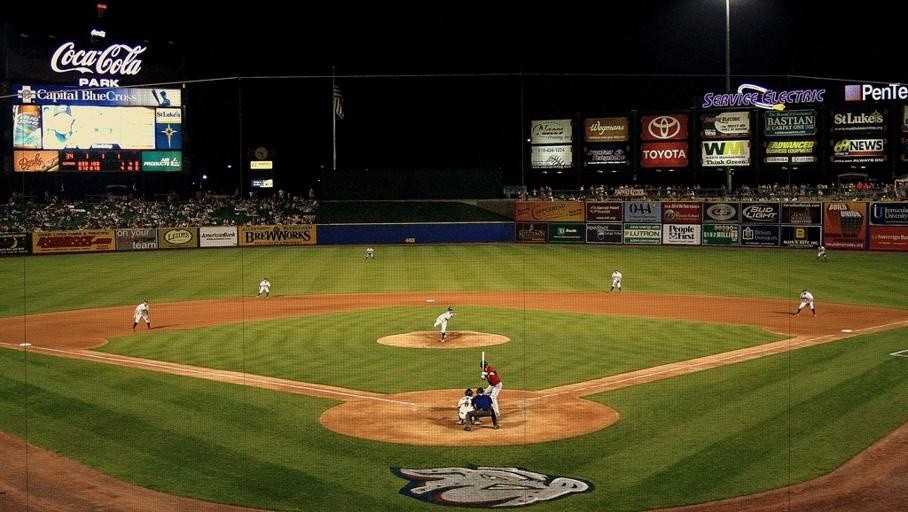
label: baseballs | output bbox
[454,317,456,318]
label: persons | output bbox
[256,277,271,298]
[464,387,499,430]
[795,288,816,317]
[365,245,376,262]
[514,177,907,203]
[455,389,481,425]
[0,183,323,234]
[610,270,622,292]
[132,299,152,331]
[159,91,171,107]
[816,245,828,262]
[480,361,503,417]
[434,307,454,343]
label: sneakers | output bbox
[456,420,481,430]
[494,413,500,429]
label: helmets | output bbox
[480,362,487,367]
[477,387,484,392]
[465,389,472,396]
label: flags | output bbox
[333,77,346,120]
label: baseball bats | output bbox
[482,351,484,372]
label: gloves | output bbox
[481,372,488,379]
